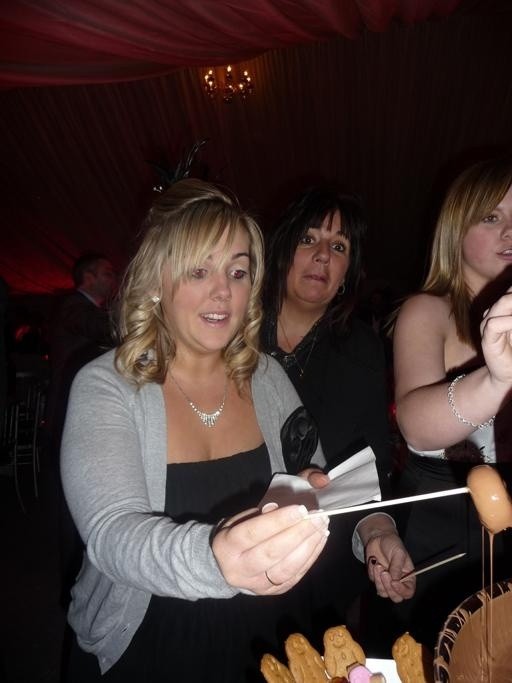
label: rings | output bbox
[265,571,282,587]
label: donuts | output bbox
[466,465,512,533]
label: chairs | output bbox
[0,370,48,520]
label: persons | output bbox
[260,187,419,665]
[59,176,331,683]
[44,249,123,614]
[383,159,512,659]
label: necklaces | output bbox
[279,316,320,377]
[169,366,228,428]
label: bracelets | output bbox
[448,373,496,431]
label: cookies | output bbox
[261,624,386,683]
[392,632,427,682]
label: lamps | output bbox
[203,64,257,103]
